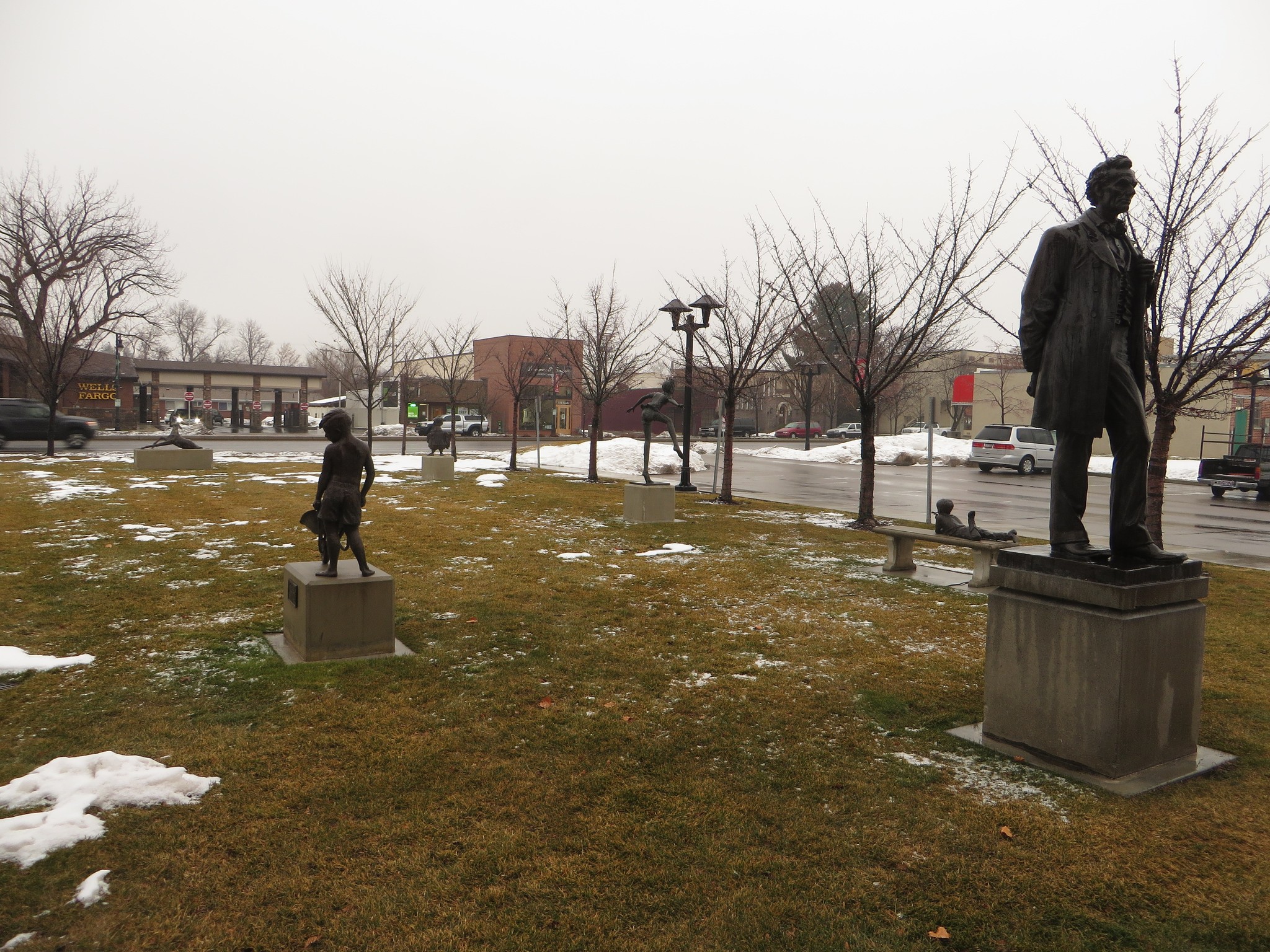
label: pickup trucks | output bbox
[1196,442,1270,501]
[414,413,490,438]
[901,421,952,437]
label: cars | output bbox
[826,422,862,439]
[169,408,200,428]
[164,409,175,424]
[198,408,224,425]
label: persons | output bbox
[1019,154,1189,564]
[935,499,1018,543]
[625,378,685,483]
[313,408,375,577]
[427,417,447,456]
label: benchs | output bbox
[873,526,1021,588]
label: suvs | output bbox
[698,416,756,438]
[968,423,1058,475]
[0,396,100,451]
[775,420,822,439]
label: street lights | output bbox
[794,358,828,450]
[658,294,725,493]
[113,332,125,432]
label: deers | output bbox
[136,421,204,450]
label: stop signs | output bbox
[300,402,309,411]
[203,400,212,409]
[184,391,195,402]
[253,401,262,410]
